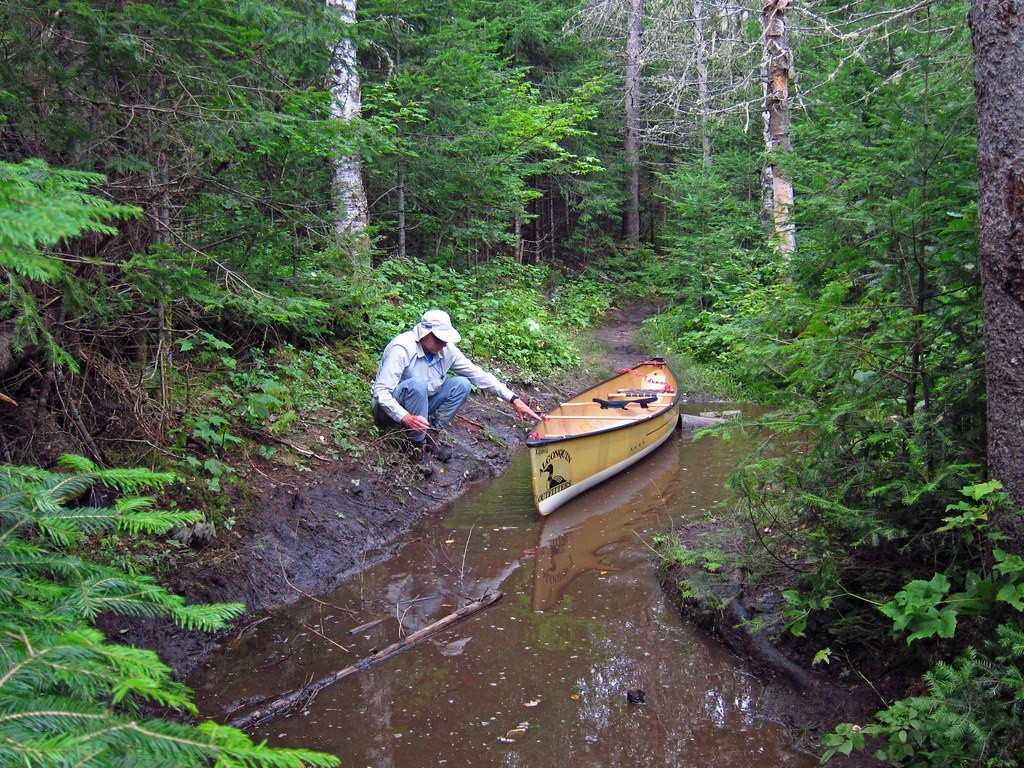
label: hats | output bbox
[413,310,462,344]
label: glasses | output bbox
[430,333,448,344]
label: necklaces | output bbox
[426,355,445,379]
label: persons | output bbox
[370,310,541,475]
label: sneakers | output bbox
[405,443,433,477]
[425,431,453,460]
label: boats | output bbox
[525,356,682,517]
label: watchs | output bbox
[510,394,519,404]
